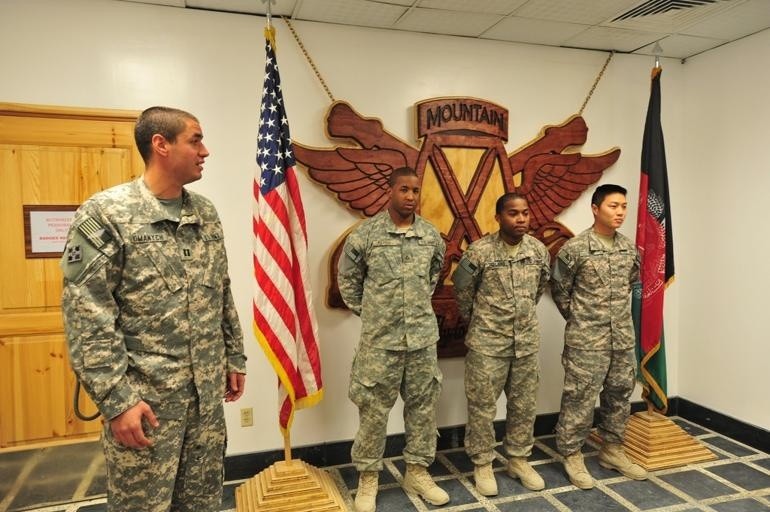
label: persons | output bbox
[336,166,450,512]
[58,106,248,511]
[452,192,551,497]
[548,184,648,490]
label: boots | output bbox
[561,448,594,489]
[354,471,379,512]
[473,461,498,496]
[507,456,545,491]
[402,462,450,506]
[598,440,649,481]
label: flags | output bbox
[252,24,325,441]
[631,65,677,416]
